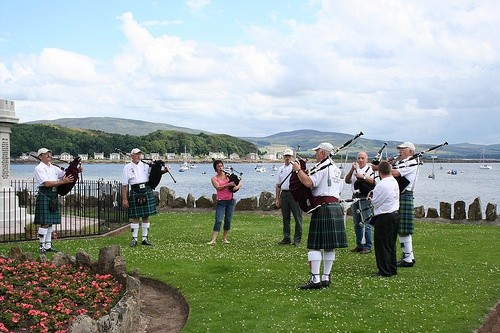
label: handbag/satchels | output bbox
[136,195,147,205]
[49,199,59,212]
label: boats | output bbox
[255,163,267,173]
[428,155,435,179]
[447,168,457,174]
[480,164,492,169]
[270,164,278,171]
[179,162,197,173]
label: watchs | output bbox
[296,169,301,174]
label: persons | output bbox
[275,149,304,247]
[345,152,377,254]
[122,148,171,247]
[370,161,400,276]
[293,143,349,289]
[207,160,243,246]
[391,141,420,267]
[33,148,84,253]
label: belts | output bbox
[281,190,291,192]
[42,185,57,192]
[131,182,150,189]
[394,210,399,213]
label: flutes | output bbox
[276,132,364,188]
[25,153,83,196]
[205,152,244,193]
[353,165,381,181]
[372,141,449,170]
[113,148,176,182]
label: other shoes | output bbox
[277,237,291,244]
[222,239,230,244]
[207,241,216,246]
[350,246,362,251]
[362,248,373,254]
[292,241,300,246]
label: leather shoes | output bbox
[141,239,150,245]
[46,248,61,253]
[397,258,417,267]
[130,240,138,247]
[40,247,46,254]
[300,281,321,290]
[372,272,390,277]
[321,279,330,287]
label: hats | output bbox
[37,148,51,156]
[130,148,141,154]
[312,142,334,154]
[284,149,294,156]
[397,142,416,152]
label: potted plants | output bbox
[26,223,41,238]
